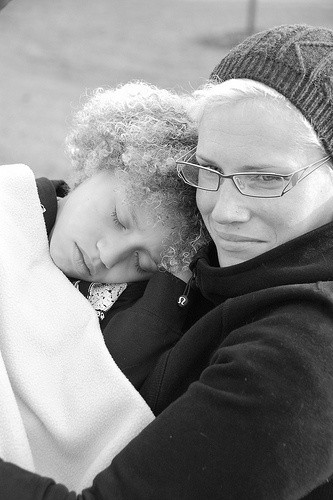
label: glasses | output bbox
[175,146,331,199]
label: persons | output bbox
[1,78,210,339]
[0,25,333,500]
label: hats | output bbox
[209,24,333,164]
